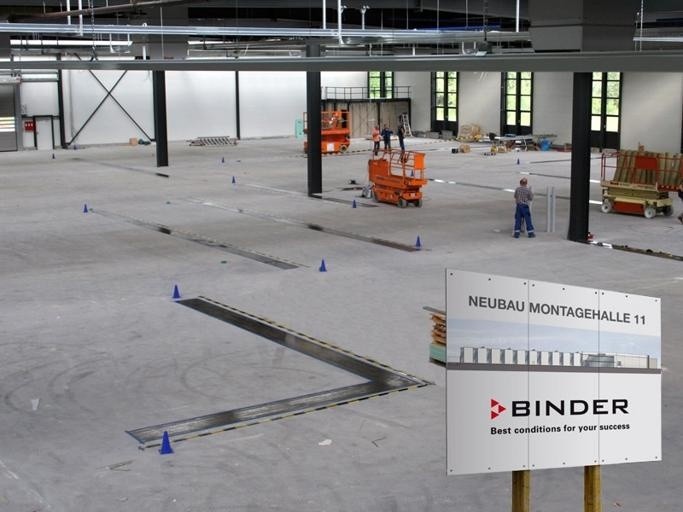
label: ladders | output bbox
[186,136,234,147]
[401,114,412,137]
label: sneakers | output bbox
[513,232,521,238]
[528,232,536,238]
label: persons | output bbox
[513,178,536,238]
[372,124,393,156]
[398,125,405,150]
